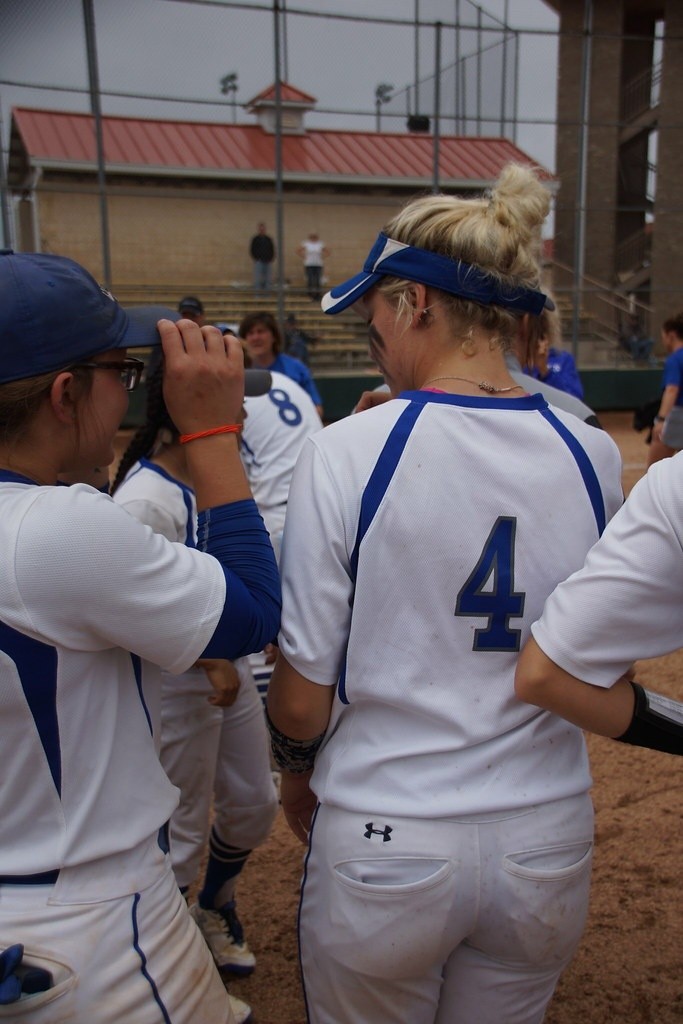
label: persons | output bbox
[647,312,683,471]
[250,221,275,291]
[265,160,624,1024]
[295,229,329,287]
[522,284,584,399]
[238,310,323,416]
[515,451,683,755]
[219,327,324,705]
[503,296,602,430]
[179,298,205,325]
[111,338,278,974]
[279,313,322,372]
[0,248,282,1024]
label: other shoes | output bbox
[227,994,253,1024]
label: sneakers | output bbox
[188,899,257,976]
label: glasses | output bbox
[75,358,145,389]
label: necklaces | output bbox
[423,377,523,393]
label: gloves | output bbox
[0,943,50,1006]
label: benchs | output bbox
[100,284,599,370]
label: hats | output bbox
[1,249,183,387]
[320,231,547,317]
[179,298,201,315]
[148,346,272,411]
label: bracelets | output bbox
[178,424,239,444]
[656,415,665,422]
